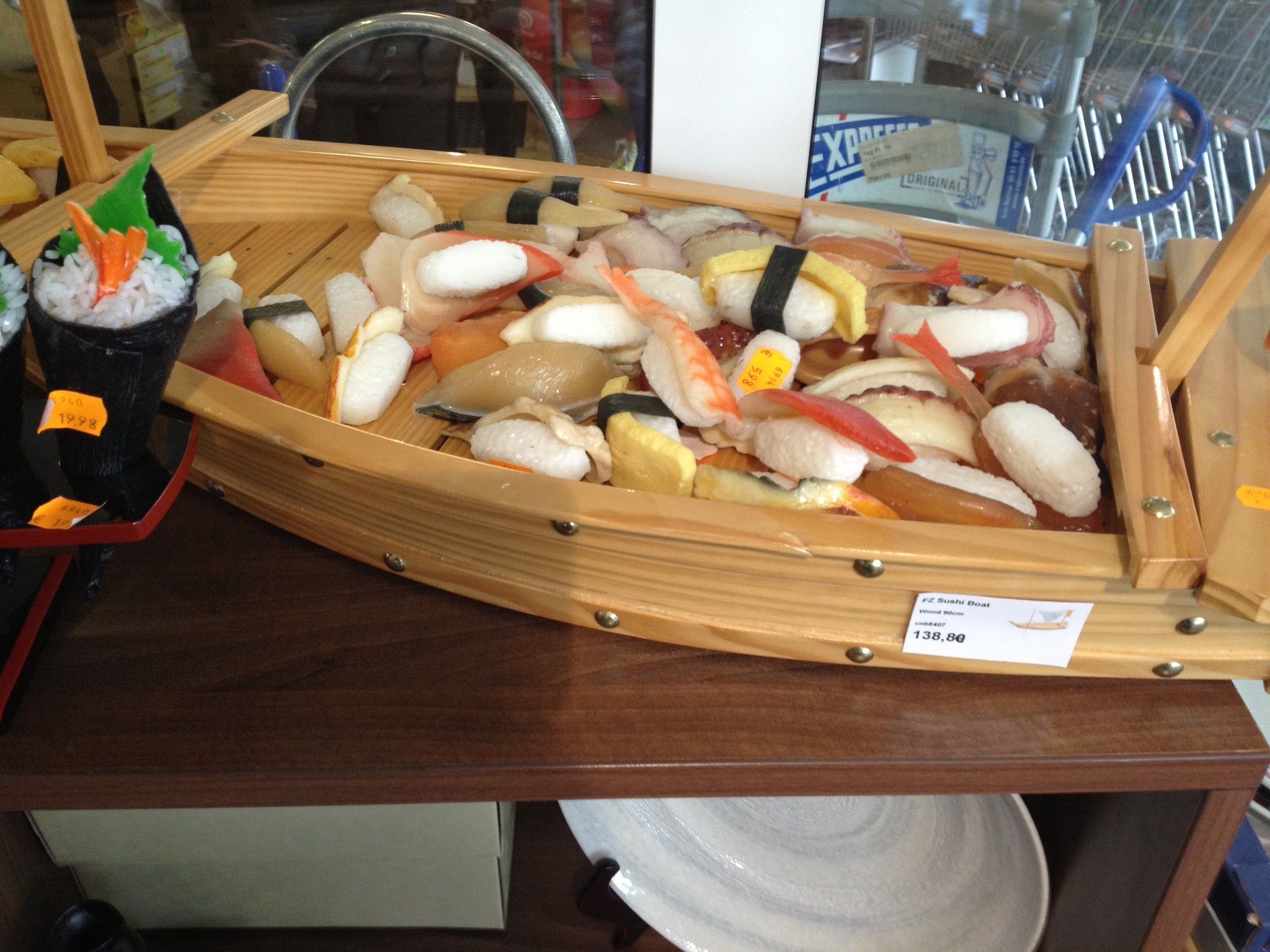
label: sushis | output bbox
[170,170,1109,537]
[0,136,203,472]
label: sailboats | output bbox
[3,0,1270,683]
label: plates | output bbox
[559,793,1050,952]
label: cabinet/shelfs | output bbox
[0,481,1270,952]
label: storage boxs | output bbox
[116,0,199,126]
[28,801,516,929]
